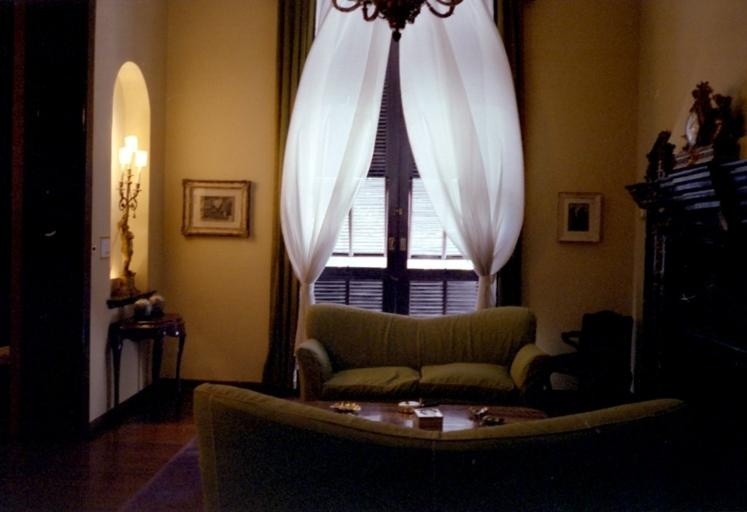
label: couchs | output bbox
[296,303,550,404]
[193,382,686,512]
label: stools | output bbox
[104,313,187,416]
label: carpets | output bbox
[115,433,203,512]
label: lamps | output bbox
[114,135,148,298]
[332,0,463,43]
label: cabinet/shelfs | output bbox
[622,158,747,512]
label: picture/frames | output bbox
[556,192,603,242]
[181,179,252,238]
